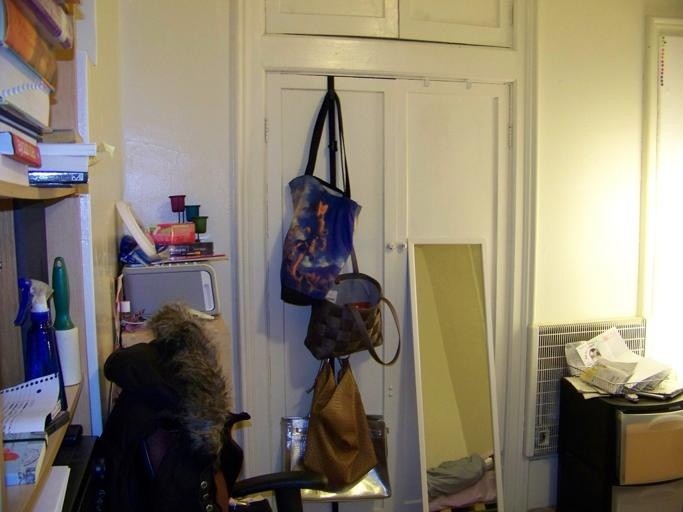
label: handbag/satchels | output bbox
[300,354,378,487]
[304,247,401,366]
[280,415,392,502]
[280,91,362,306]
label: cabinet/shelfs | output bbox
[118,315,234,419]
[555,376,683,512]
[0,2,101,512]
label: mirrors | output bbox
[406,237,504,512]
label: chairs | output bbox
[141,414,329,512]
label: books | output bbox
[0,0,97,192]
[44,396,65,425]
[637,379,683,400]
[159,242,231,264]
[4,431,49,487]
